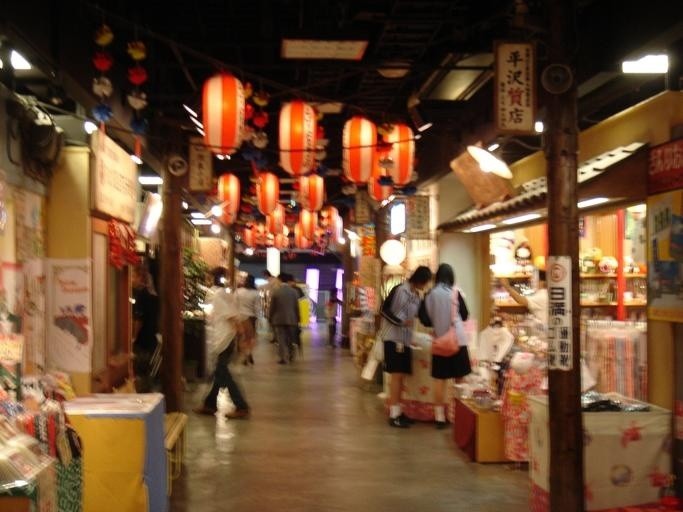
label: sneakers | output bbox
[389,412,447,430]
[191,404,250,418]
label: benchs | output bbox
[111,380,188,496]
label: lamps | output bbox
[465,135,547,180]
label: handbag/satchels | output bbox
[431,326,460,358]
[374,330,387,363]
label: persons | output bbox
[132,252,306,419]
[379,265,432,427]
[501,269,550,335]
[327,288,343,348]
[419,264,473,429]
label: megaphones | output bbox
[167,155,189,177]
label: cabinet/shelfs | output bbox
[492,272,646,309]
